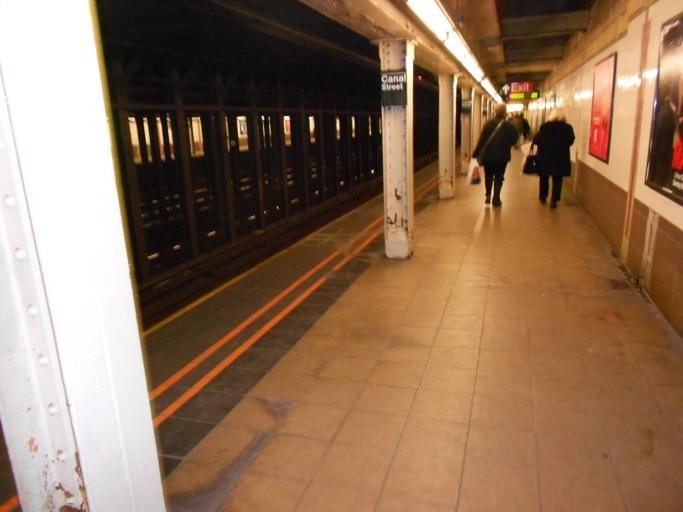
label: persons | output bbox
[472,103,518,206]
[533,107,575,209]
[508,111,529,144]
[648,97,677,188]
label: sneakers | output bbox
[539,197,557,208]
[485,196,502,207]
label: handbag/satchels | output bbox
[523,155,536,174]
[477,148,485,166]
